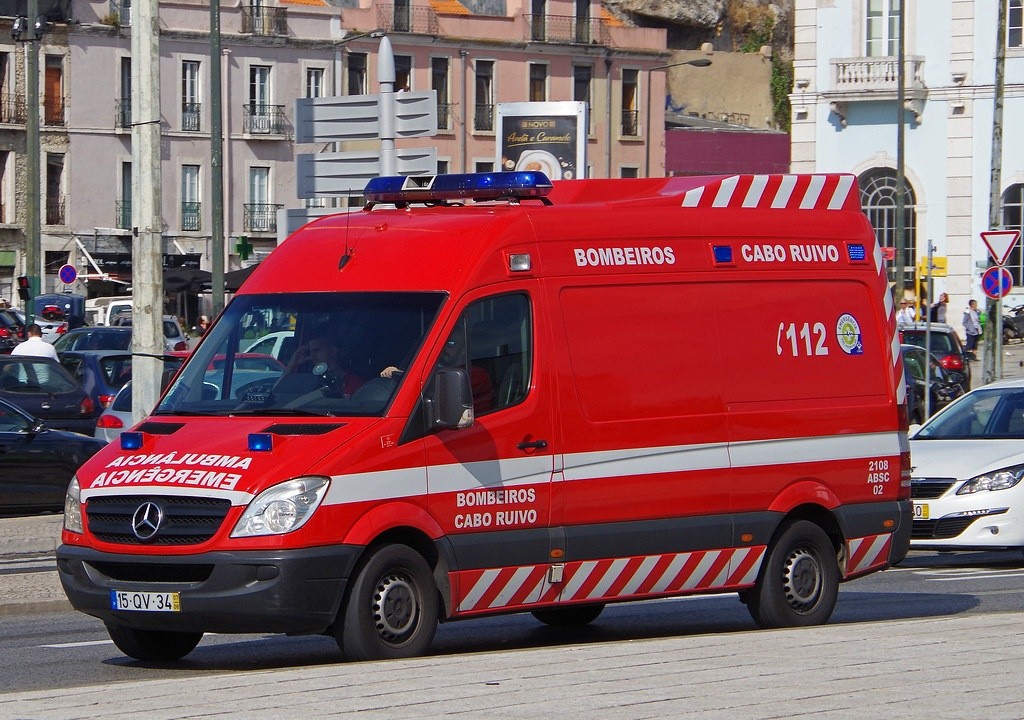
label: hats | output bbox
[320,374,365,398]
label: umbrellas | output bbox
[199,262,262,293]
[165,263,212,326]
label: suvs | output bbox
[899,322,973,395]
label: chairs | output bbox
[469,319,522,408]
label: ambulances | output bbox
[53,171,919,666]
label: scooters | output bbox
[1002,304,1024,345]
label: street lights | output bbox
[331,29,386,208]
[646,59,714,181]
[11,14,46,328]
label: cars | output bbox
[0,354,98,438]
[909,379,1023,551]
[0,398,109,508]
[899,343,967,433]
[0,288,296,448]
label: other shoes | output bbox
[974,358,980,362]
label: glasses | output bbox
[900,303,906,304]
[448,342,464,348]
[309,347,323,354]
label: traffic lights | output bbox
[18,288,31,300]
[17,276,31,288]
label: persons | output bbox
[271,328,361,398]
[895,293,949,323]
[109,309,126,326]
[9,325,59,383]
[197,316,208,335]
[962,300,982,361]
[48,311,89,332]
[380,329,494,416]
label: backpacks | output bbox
[931,303,940,322]
